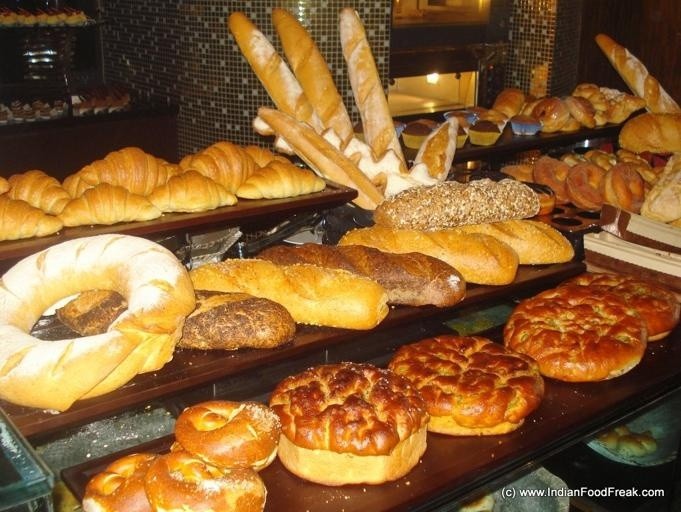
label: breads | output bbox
[0,8,681,512]
